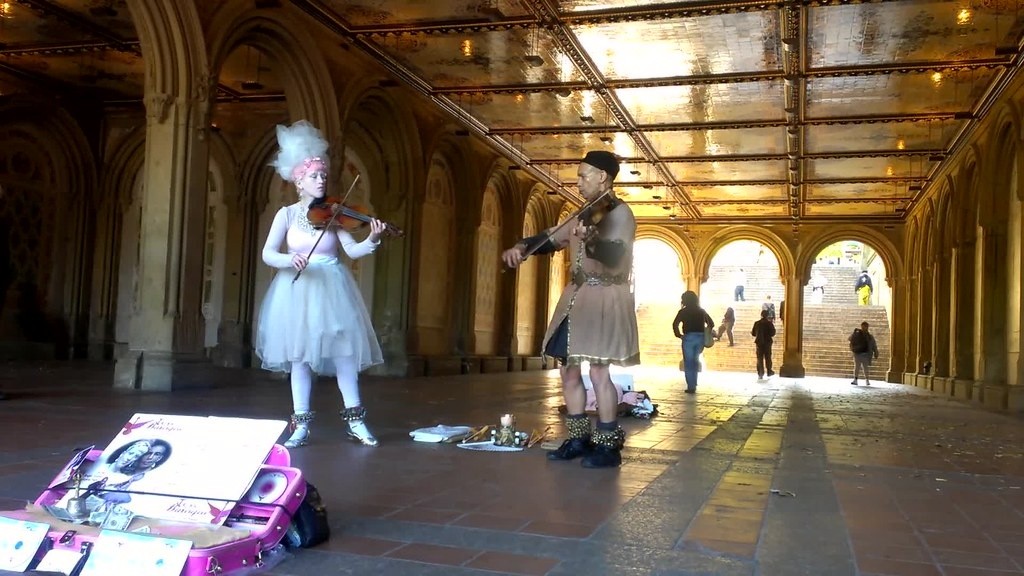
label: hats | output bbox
[581,150,620,177]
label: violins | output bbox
[578,190,616,260]
[306,195,408,240]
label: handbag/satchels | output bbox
[851,328,868,353]
[288,479,331,548]
[703,317,714,348]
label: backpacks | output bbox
[764,303,775,319]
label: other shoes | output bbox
[344,423,381,448]
[767,371,774,376]
[546,439,595,460]
[851,381,857,385]
[758,375,763,378]
[583,450,621,468]
[685,390,695,393]
[284,430,310,449]
[866,381,869,385]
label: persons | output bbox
[79,439,165,511]
[255,121,386,447]
[503,151,636,468]
[673,269,879,393]
